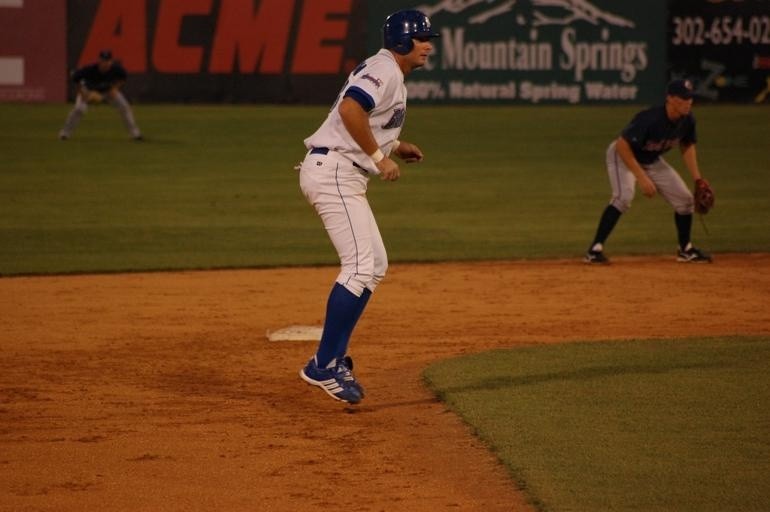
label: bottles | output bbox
[666,80,698,99]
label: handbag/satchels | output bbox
[369,148,385,164]
[391,140,401,153]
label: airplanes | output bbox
[695,180,714,216]
[83,90,101,103]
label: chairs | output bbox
[299,354,366,405]
[676,249,714,264]
[586,247,608,263]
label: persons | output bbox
[58,46,146,142]
[297,8,442,405]
[586,77,713,268]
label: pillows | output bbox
[385,10,439,55]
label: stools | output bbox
[309,147,370,173]
